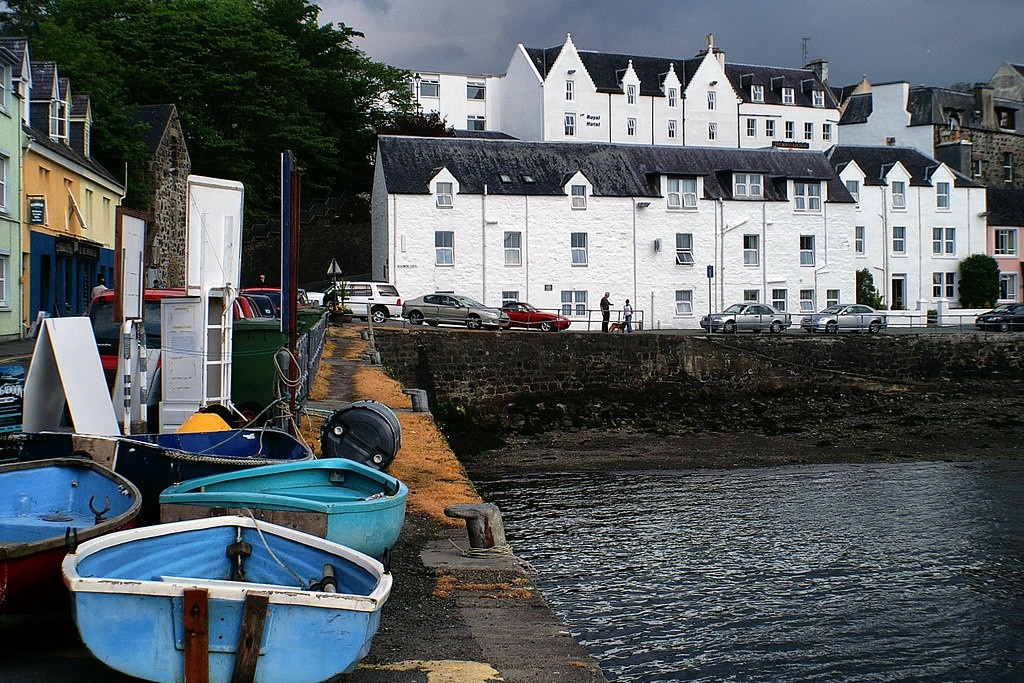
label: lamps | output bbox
[638,202,651,207]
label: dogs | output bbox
[608,321,628,333]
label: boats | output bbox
[9,424,317,518]
[158,457,408,558]
[0,448,145,608]
[60,513,396,683]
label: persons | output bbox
[92,279,108,303]
[623,299,633,334]
[259,274,269,288]
[600,292,614,333]
[152,278,163,289]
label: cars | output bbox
[700,303,793,334]
[500,303,572,331]
[973,302,1024,332]
[800,304,887,334]
[401,292,511,330]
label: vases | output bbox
[329,314,353,324]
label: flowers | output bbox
[329,304,354,314]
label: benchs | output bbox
[0,513,97,542]
[264,486,369,503]
[161,575,304,591]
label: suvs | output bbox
[83,286,311,394]
[302,280,403,324]
